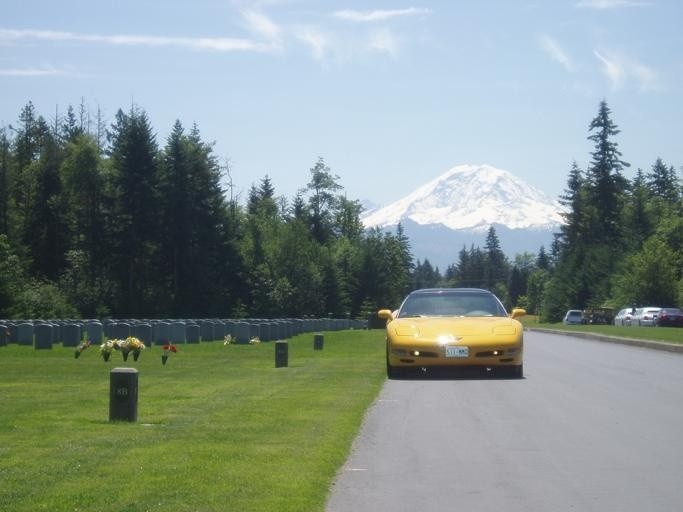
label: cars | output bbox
[563,307,683,327]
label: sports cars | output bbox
[377,288,526,379]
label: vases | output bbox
[123,353,128,361]
[133,353,140,361]
[75,351,80,359]
[103,354,109,361]
[162,356,168,365]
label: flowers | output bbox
[101,336,145,353]
[248,335,261,345]
[79,339,91,353]
[223,334,236,346]
[161,344,176,356]
